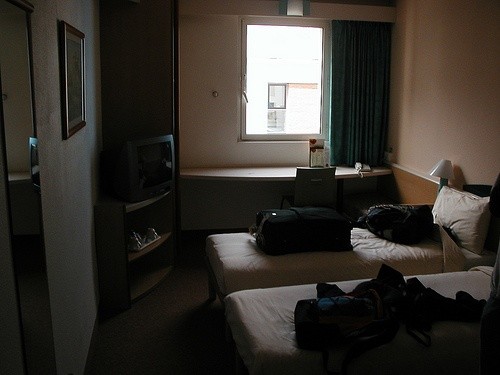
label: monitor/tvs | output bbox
[100,134,175,203]
[29,137,40,193]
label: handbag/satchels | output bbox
[353,264,487,350]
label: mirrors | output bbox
[1,0,59,375]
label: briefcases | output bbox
[295,295,391,375]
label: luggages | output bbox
[256,208,353,256]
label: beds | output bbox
[223,265,494,375]
[202,183,500,338]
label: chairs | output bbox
[279,166,337,210]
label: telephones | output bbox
[355,161,372,172]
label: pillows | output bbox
[428,185,493,257]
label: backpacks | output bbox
[366,205,433,245]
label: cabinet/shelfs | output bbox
[94,189,176,310]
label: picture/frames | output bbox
[57,19,87,141]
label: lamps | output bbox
[427,160,454,192]
